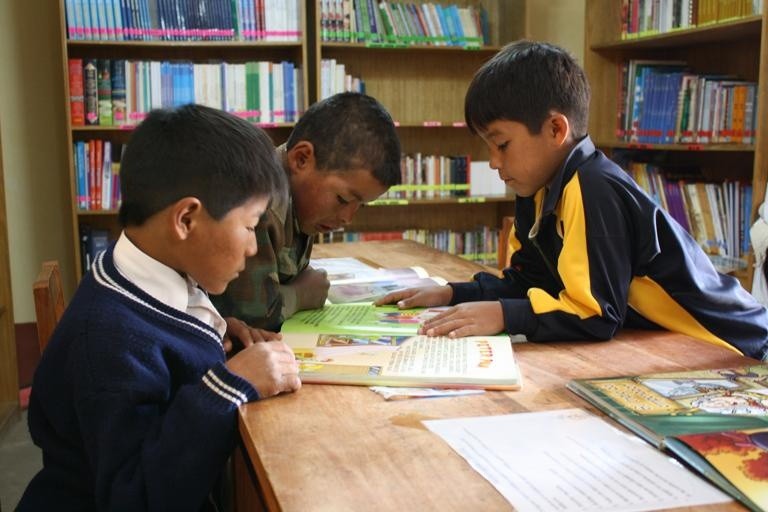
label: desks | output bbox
[237,240,767,512]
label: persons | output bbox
[13,104,304,511]
[373,39,768,371]
[195,92,403,358]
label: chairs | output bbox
[35,261,68,356]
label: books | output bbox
[318,1,510,265]
[326,265,451,304]
[565,364,768,512]
[270,303,524,394]
[614,0,768,277]
[65,1,299,274]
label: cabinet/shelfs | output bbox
[580,1,766,295]
[58,0,531,284]
